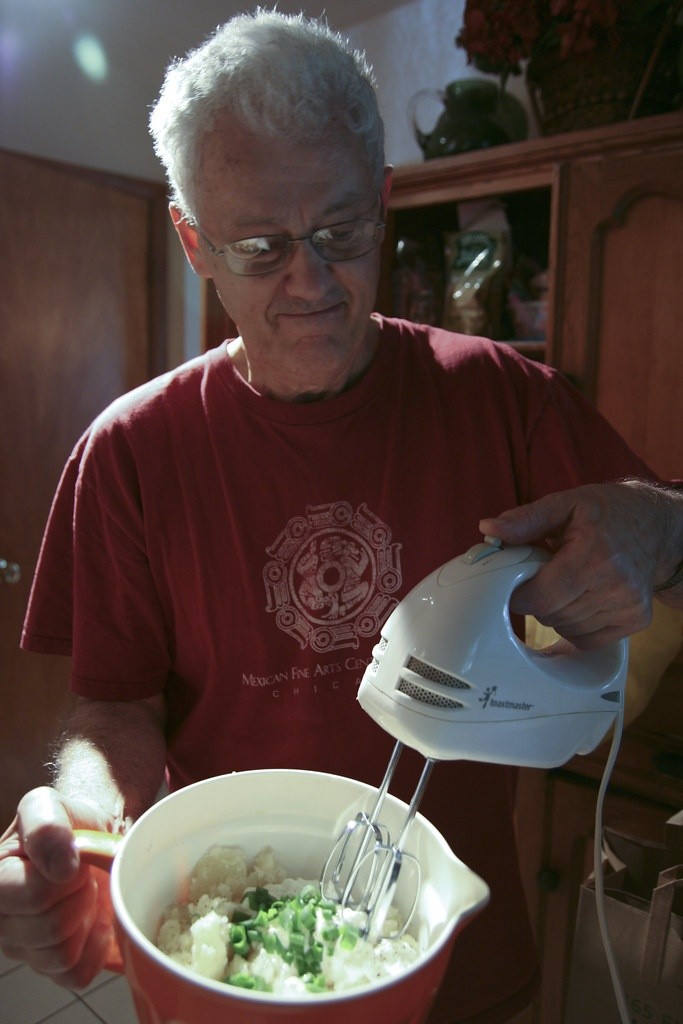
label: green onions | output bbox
[220,878,361,994]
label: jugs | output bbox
[69,768,494,1024]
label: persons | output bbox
[0,4,683,1024]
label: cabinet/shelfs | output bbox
[198,111,683,1024]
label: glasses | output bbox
[193,193,386,277]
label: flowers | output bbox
[454,0,663,75]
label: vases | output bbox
[527,19,683,130]
